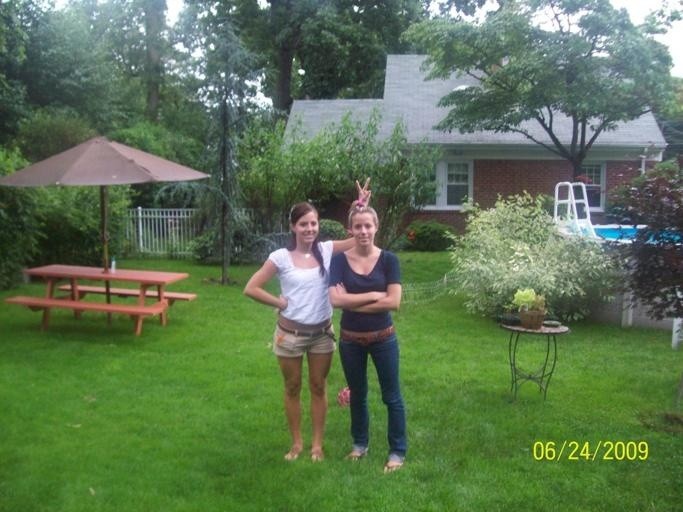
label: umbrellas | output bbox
[0,136,213,273]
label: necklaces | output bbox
[303,253,311,258]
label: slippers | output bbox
[311,447,324,462]
[344,450,368,459]
[284,446,303,461]
[384,459,403,474]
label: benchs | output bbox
[3,283,198,335]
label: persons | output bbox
[243,177,373,464]
[328,199,407,474]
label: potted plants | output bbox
[512,287,547,333]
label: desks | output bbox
[23,264,189,336]
[495,321,570,406]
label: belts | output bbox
[277,321,332,336]
[340,324,395,345]
